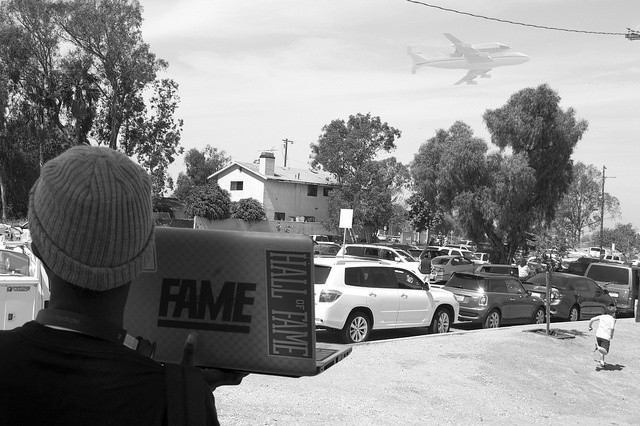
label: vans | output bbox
[336,244,428,282]
[590,246,600,258]
[310,234,329,243]
[584,263,640,317]
[603,255,621,263]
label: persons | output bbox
[590,306,618,366]
[421,253,431,282]
[1,146,252,426]
[594,290,617,316]
[518,259,529,276]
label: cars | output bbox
[527,257,567,271]
[519,272,617,321]
[429,255,481,283]
[474,252,488,261]
[442,270,547,329]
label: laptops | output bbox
[123,226,352,378]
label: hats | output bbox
[28,145,155,291]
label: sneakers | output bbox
[599,361,607,366]
[593,343,597,351]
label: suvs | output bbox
[313,242,342,255]
[419,246,487,270]
[313,256,460,343]
